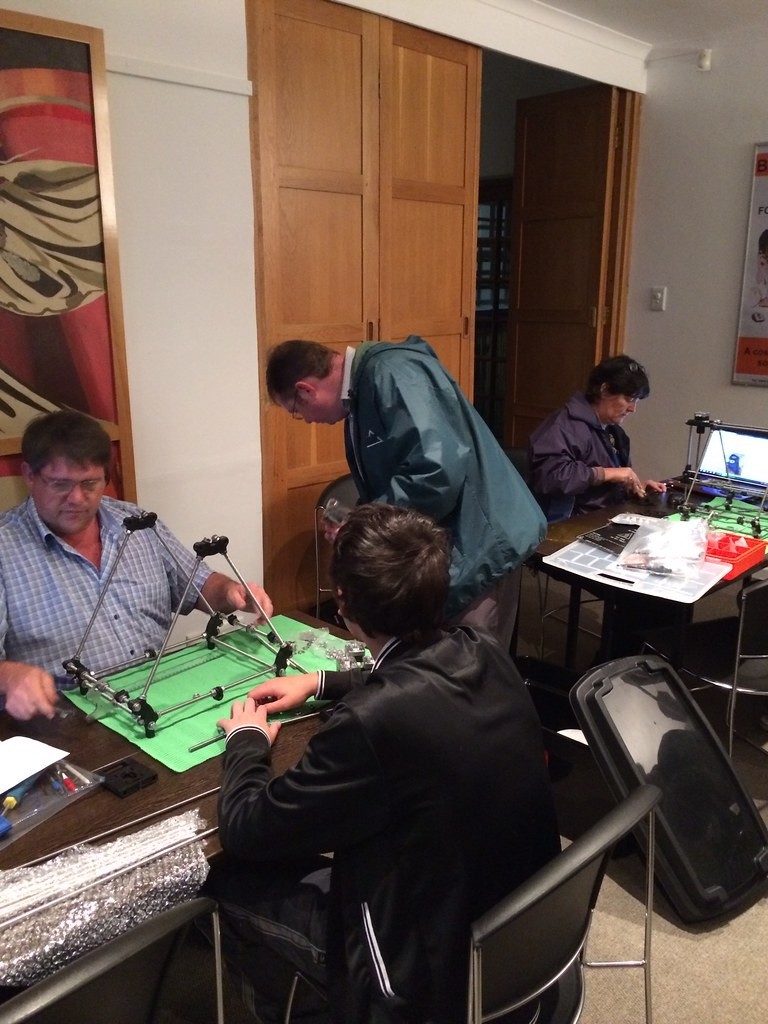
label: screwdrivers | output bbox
[0,769,44,817]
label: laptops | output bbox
[659,422,768,501]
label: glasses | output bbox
[291,390,304,420]
[34,468,106,495]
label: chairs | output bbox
[285,783,665,1024]
[505,447,604,658]
[0,897,224,1024]
[637,578,768,759]
[315,473,361,619]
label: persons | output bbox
[266,335,547,652]
[0,409,274,721]
[192,502,562,1024]
[751,229,768,307]
[527,356,667,666]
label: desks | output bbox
[0,609,356,981]
[526,476,768,680]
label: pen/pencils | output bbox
[58,771,75,790]
[49,777,64,793]
[65,764,90,784]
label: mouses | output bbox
[668,494,685,504]
